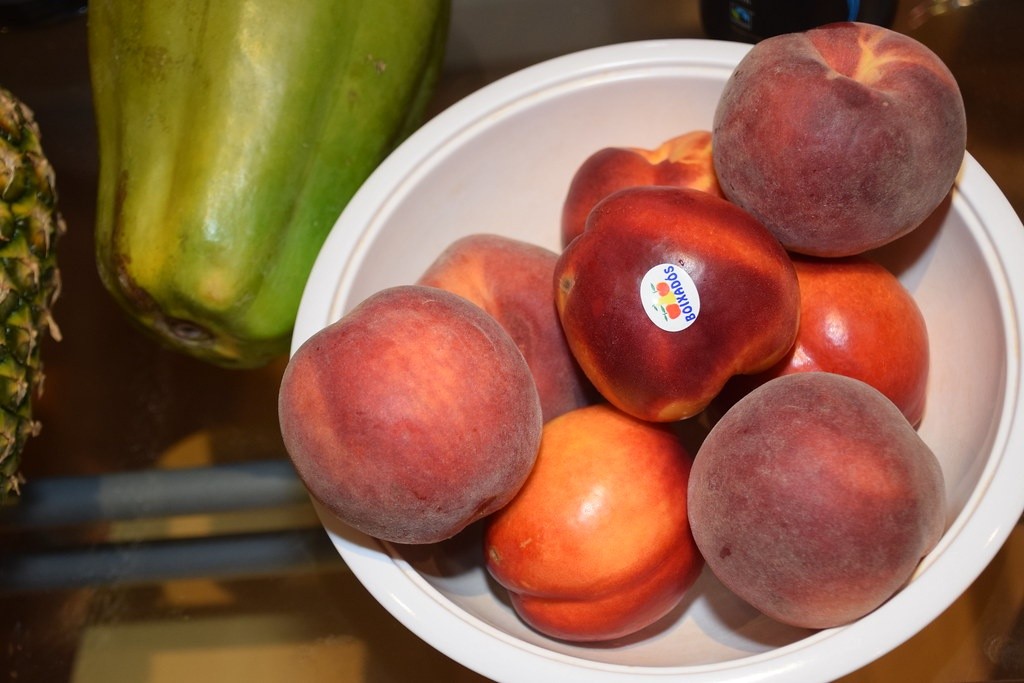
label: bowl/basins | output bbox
[289,38,1024,683]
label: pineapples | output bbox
[0,88,68,519]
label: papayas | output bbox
[81,1,455,367]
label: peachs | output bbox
[274,21,970,641]
